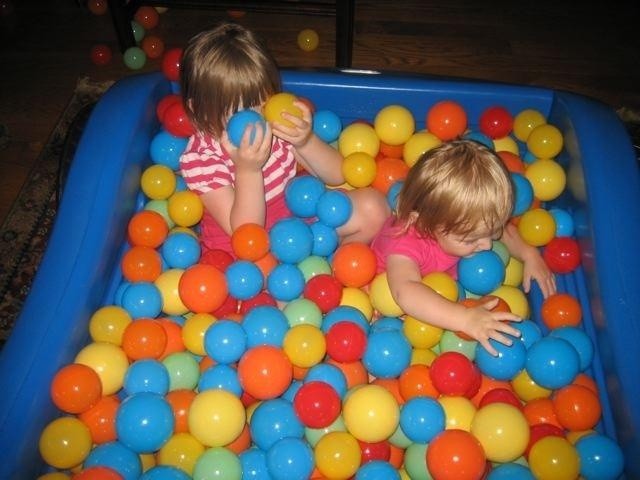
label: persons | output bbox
[371,136,558,358]
[179,23,391,263]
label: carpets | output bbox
[0,76,117,357]
